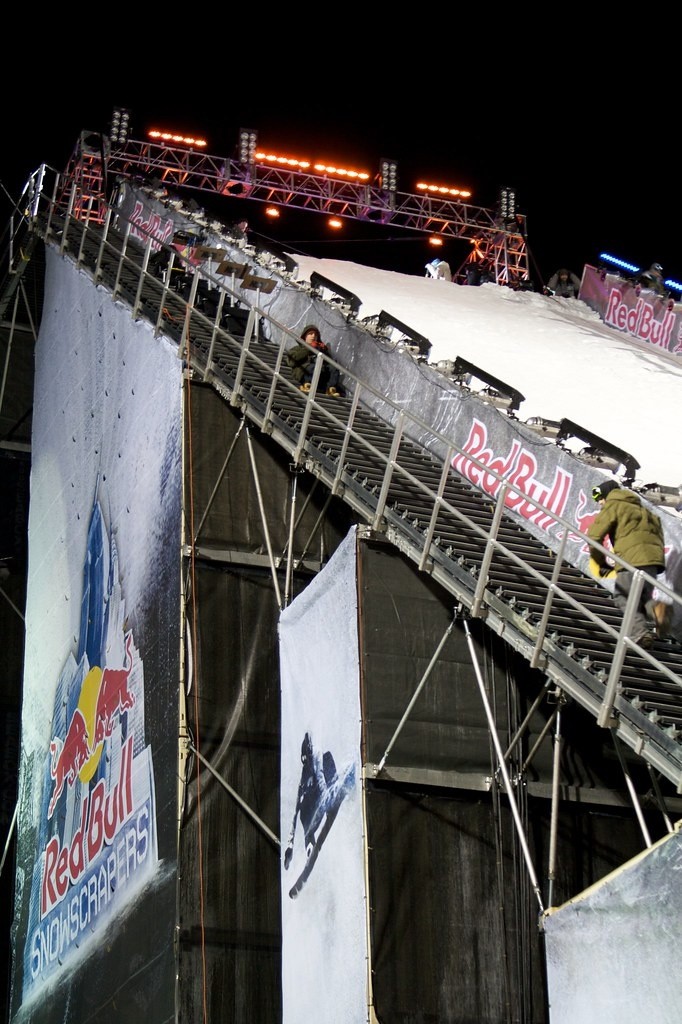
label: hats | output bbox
[595,479,621,502]
[299,731,313,764]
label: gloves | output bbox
[597,560,614,578]
[310,340,327,351]
[284,848,294,870]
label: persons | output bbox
[285,325,342,399]
[424,259,452,281]
[543,269,580,297]
[284,732,336,880]
[587,479,674,649]
[233,220,249,243]
[635,263,663,295]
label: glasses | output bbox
[591,486,601,499]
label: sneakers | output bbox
[626,633,653,654]
[654,602,674,640]
[305,843,314,858]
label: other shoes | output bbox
[299,382,312,392]
[325,386,340,397]
